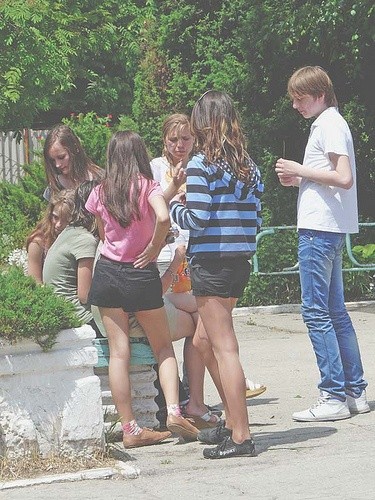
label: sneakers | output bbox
[203,436,255,459]
[291,397,351,422]
[345,389,370,414]
[198,427,233,444]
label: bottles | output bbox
[172,256,191,293]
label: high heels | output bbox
[245,375,266,398]
[184,403,220,428]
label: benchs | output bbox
[93,337,160,442]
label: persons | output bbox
[168,87,264,459]
[25,114,267,448]
[274,67,370,423]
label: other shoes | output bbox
[186,401,223,418]
[166,414,199,442]
[123,427,171,448]
